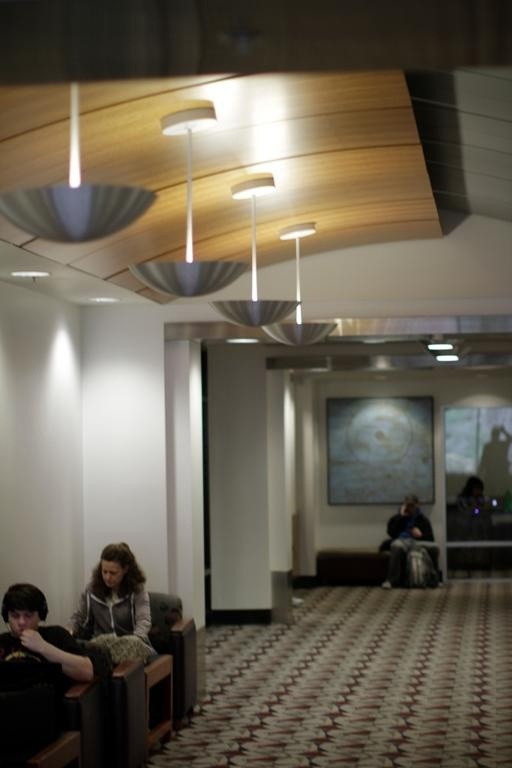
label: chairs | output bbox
[25,591,197,765]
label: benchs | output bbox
[315,548,437,587]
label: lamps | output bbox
[127,122,250,299]
[209,196,302,328]
[0,82,158,247]
[261,237,339,347]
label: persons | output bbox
[456,476,493,578]
[381,493,444,590]
[0,582,107,768]
[475,425,512,499]
[64,542,158,666]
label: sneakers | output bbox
[381,579,392,590]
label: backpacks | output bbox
[406,548,439,587]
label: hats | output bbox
[404,494,418,506]
